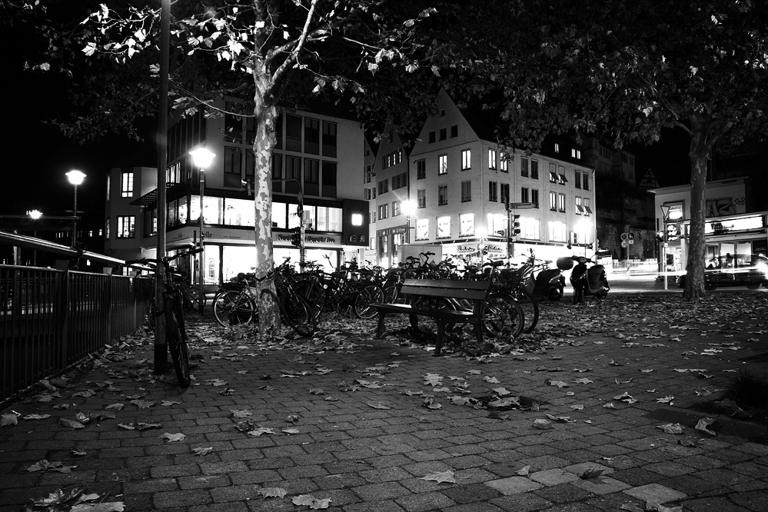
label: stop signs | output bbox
[297,204,304,217]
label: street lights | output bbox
[400,200,417,243]
[476,225,487,272]
[65,169,86,245]
[581,220,588,257]
[189,147,216,308]
[240,179,305,261]
[660,203,670,290]
[514,215,520,236]
[29,209,42,266]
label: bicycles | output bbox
[125,248,206,388]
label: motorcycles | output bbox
[535,255,611,305]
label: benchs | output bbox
[366,275,494,357]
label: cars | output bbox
[676,253,768,290]
